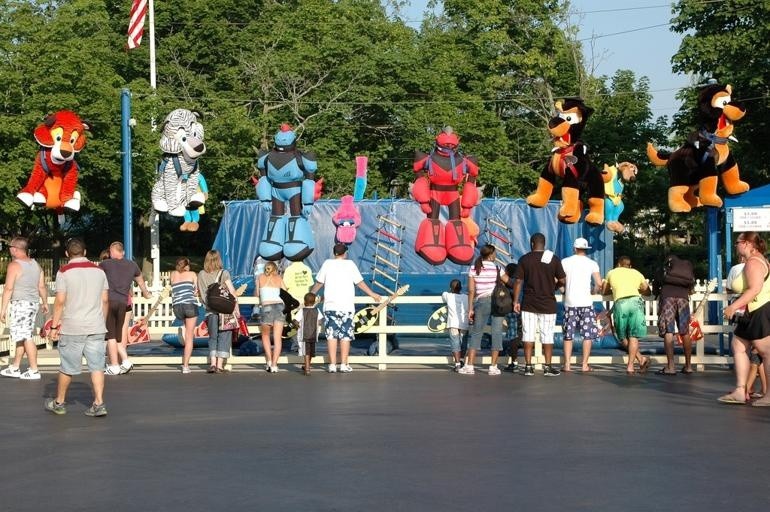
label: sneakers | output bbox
[82,402,108,417]
[264,361,353,375]
[105,360,133,375]
[45,397,66,415]
[454,362,560,376]
[0,366,42,379]
[181,366,229,374]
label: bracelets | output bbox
[50,325,57,330]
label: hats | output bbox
[334,244,348,256]
[573,238,592,250]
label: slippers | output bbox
[626,358,694,375]
[716,388,770,407]
[562,366,594,372]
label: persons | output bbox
[98,241,152,374]
[44,237,111,417]
[438,232,696,379]
[311,243,381,373]
[714,230,770,407]
[196,249,242,375]
[725,252,767,399]
[290,291,327,377]
[99,250,135,362]
[170,257,199,375]
[1,237,48,380]
[282,260,316,352]
[253,261,287,374]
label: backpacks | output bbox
[654,257,696,287]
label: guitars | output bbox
[281,295,322,338]
[353,283,410,335]
[128,284,172,345]
[677,277,718,346]
[194,283,248,338]
[595,302,614,338]
[428,304,447,332]
[40,314,62,341]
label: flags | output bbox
[125,1,147,50]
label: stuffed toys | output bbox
[645,83,749,213]
[600,161,639,234]
[250,123,324,262]
[332,195,361,245]
[18,109,90,224]
[152,109,210,232]
[525,97,606,226]
[413,126,480,266]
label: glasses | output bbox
[733,240,745,246]
[7,244,15,249]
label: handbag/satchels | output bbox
[731,296,750,324]
[208,283,236,313]
[493,283,513,317]
